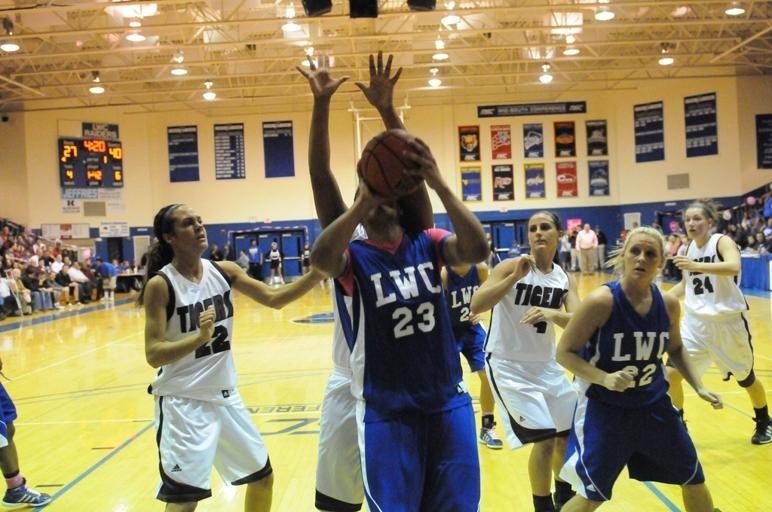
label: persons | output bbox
[559,221,609,278]
[0,224,142,322]
[264,236,287,286]
[294,49,434,512]
[667,187,772,446]
[235,249,251,274]
[132,202,329,512]
[209,238,236,262]
[469,209,582,512]
[662,218,688,281]
[555,225,721,511]
[300,242,313,276]
[439,260,505,451]
[309,136,491,511]
[247,237,264,281]
[0,356,53,507]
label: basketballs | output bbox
[358,128,425,199]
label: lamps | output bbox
[348,0,380,19]
[404,0,437,15]
[300,1,332,19]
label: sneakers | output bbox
[751,418,772,446]
[480,425,504,450]
[2,479,51,508]
